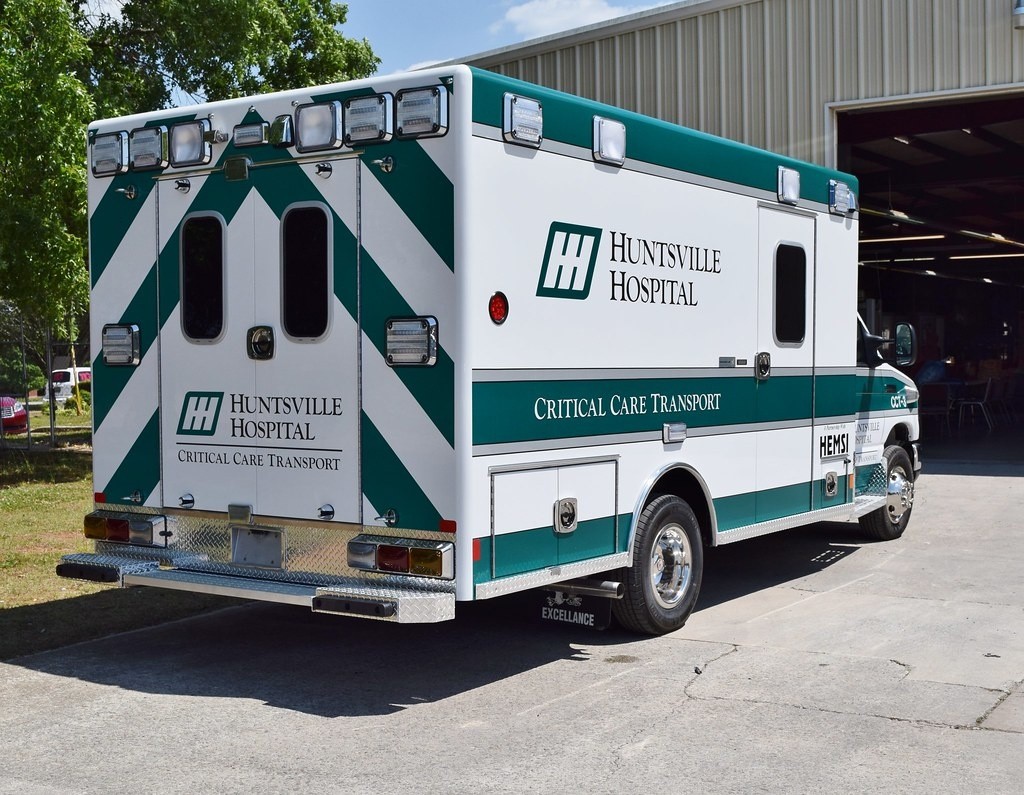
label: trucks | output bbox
[53,63,925,638]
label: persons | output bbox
[900,337,908,354]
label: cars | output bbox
[0,396,28,435]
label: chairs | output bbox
[919,383,953,440]
[956,376,1002,435]
[987,376,1021,426]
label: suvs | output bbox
[42,367,93,406]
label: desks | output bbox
[919,378,982,439]
